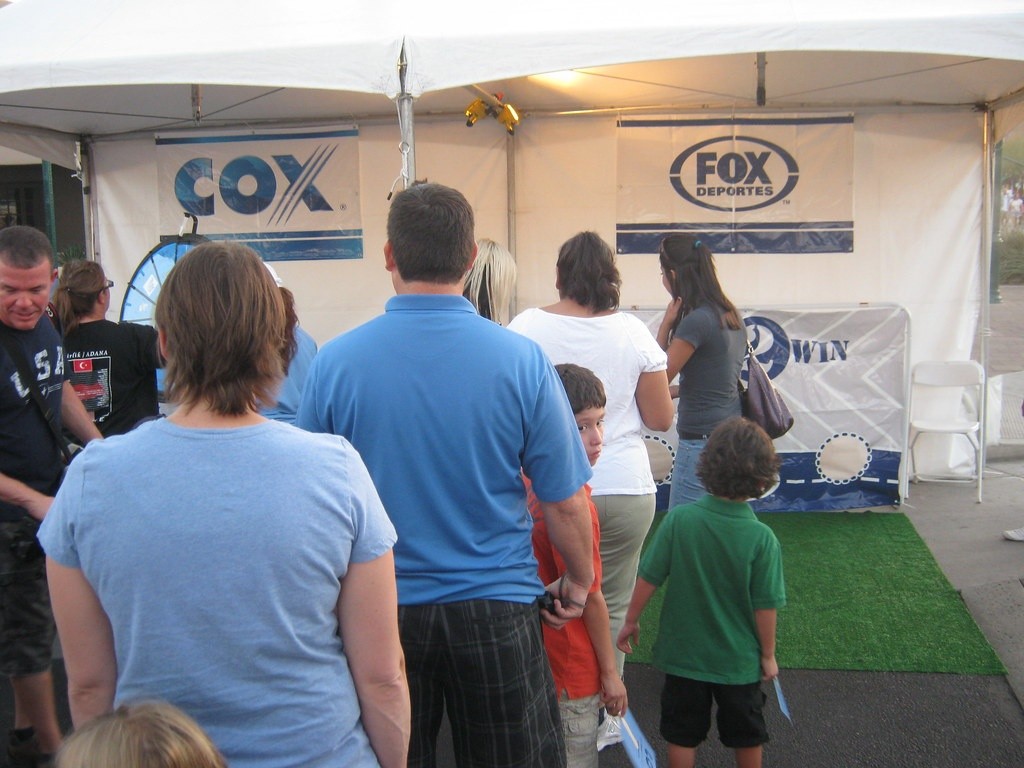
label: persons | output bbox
[509,232,675,686]
[1002,174,1024,233]
[52,260,167,448]
[34,243,411,768]
[0,225,102,768]
[56,703,220,768]
[520,364,627,768]
[254,286,318,426]
[463,238,517,326]
[656,232,747,512]
[293,184,595,768]
[616,415,786,768]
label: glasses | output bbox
[93,280,114,294]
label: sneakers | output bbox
[597,707,623,752]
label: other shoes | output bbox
[6,725,67,758]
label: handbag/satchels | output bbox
[736,339,794,439]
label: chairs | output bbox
[903,360,984,505]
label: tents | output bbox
[0,0,1024,505]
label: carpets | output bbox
[620,510,1009,677]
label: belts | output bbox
[679,432,710,440]
